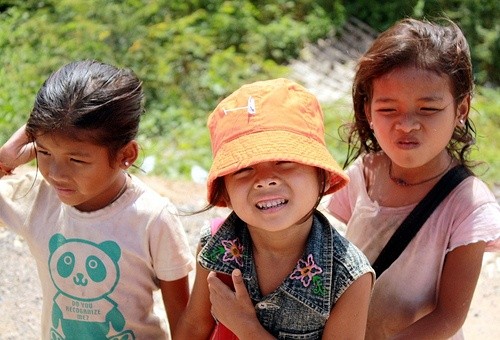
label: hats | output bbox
[206,78,349,208]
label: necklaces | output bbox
[389,158,453,186]
[107,173,129,206]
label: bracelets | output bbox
[0,162,15,177]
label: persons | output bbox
[317,19,500,340]
[172,77,375,340]
[0,60,194,340]
